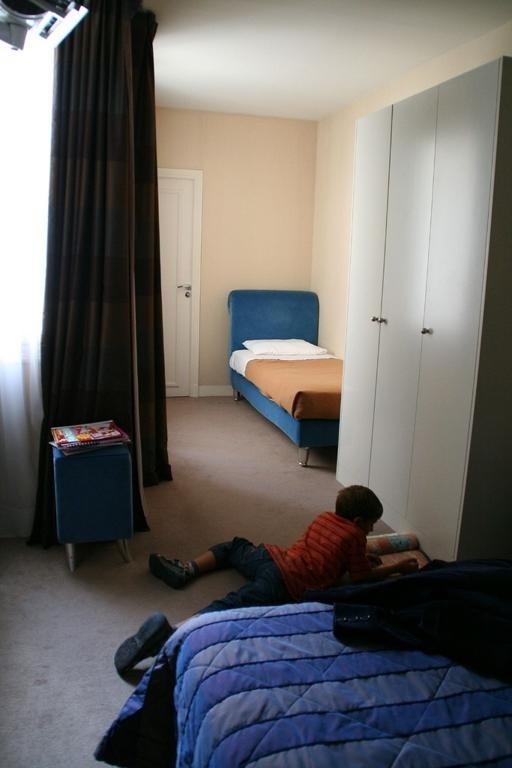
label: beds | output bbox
[228,288,344,466]
[93,600,511,768]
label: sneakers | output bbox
[147,552,193,591]
[113,613,173,674]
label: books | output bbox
[48,419,132,457]
[365,531,430,577]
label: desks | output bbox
[52,446,134,572]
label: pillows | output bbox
[242,339,328,354]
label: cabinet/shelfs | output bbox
[336,56,512,563]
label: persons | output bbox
[113,484,420,677]
